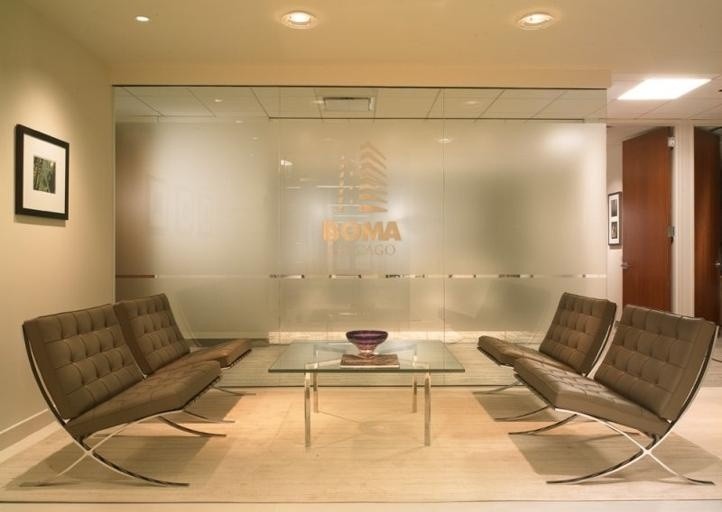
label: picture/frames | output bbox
[608,192,621,245]
[15,125,68,220]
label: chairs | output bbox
[508,305,714,485]
[473,292,618,421]
[20,304,227,487]
[115,292,256,424]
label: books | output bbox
[341,353,401,368]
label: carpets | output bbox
[0,386,721,503]
